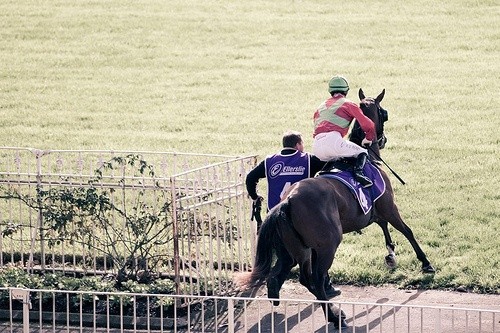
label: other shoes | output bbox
[325,283,342,298]
[266,276,280,306]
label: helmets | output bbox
[328,73,349,92]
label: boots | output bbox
[354,152,372,186]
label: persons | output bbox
[312,74,375,187]
[246,132,341,306]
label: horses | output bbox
[236,88,435,331]
